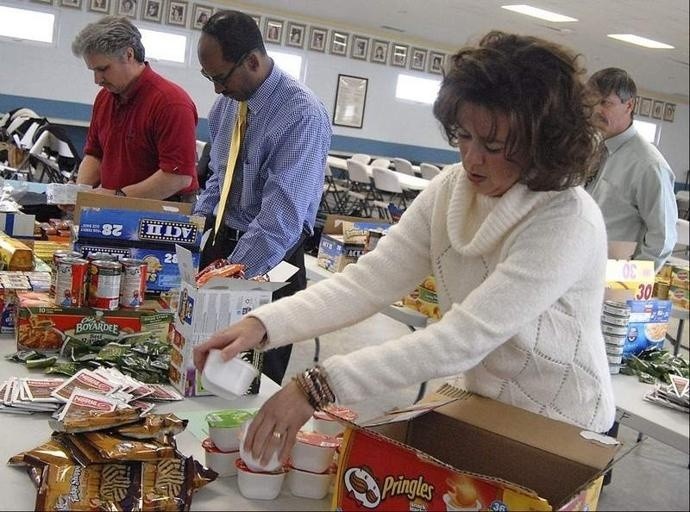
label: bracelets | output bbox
[293,363,336,410]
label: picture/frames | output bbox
[285,21,306,49]
[370,39,390,64]
[190,3,214,31]
[428,51,445,75]
[88,0,111,15]
[664,103,677,122]
[140,0,164,25]
[410,47,428,72]
[652,100,665,120]
[307,26,329,54]
[350,35,370,61]
[262,17,284,46]
[115,0,137,20]
[165,0,188,28]
[639,98,653,117]
[632,96,641,115]
[332,74,368,129]
[59,0,81,10]
[329,30,349,57]
[391,43,410,67]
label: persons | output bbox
[171,5,182,21]
[432,56,441,70]
[147,2,158,17]
[194,10,331,385]
[291,28,300,44]
[335,36,344,52]
[375,45,384,60]
[71,16,199,203]
[314,33,322,47]
[268,25,278,40]
[357,41,365,56]
[580,69,679,275]
[198,12,208,23]
[93,0,106,9]
[192,30,618,488]
[121,0,133,12]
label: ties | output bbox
[584,145,609,189]
[212,101,248,247]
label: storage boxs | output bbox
[169,244,301,397]
[623,300,673,360]
[317,215,392,273]
[71,192,207,292]
[14,292,172,354]
[319,383,648,512]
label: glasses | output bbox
[200,53,248,87]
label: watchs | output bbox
[114,188,126,198]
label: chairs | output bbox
[370,159,390,168]
[320,162,343,213]
[421,163,441,181]
[344,159,375,218]
[372,167,407,221]
[352,154,371,164]
[394,158,415,176]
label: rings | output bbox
[271,430,283,439]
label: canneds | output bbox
[48,250,84,300]
[606,344,624,356]
[603,300,632,317]
[601,311,631,327]
[54,257,89,307]
[87,260,122,311]
[85,253,117,299]
[601,322,629,336]
[119,257,148,309]
[607,354,624,365]
[603,333,626,347]
[609,363,621,375]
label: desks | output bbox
[0,331,347,512]
[428,314,690,486]
[327,155,432,191]
[303,254,429,406]
